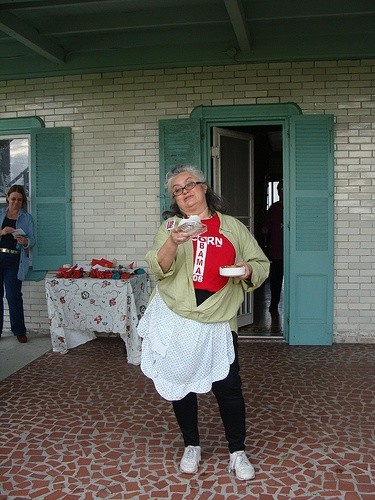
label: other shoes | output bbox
[229,450,256,479]
[179,445,202,475]
[17,335,29,344]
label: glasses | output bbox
[172,182,207,196]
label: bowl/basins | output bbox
[219,266,246,277]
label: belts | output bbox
[0,248,21,255]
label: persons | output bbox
[137,165,270,481]
[0,185,36,343]
[262,181,283,310]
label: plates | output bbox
[178,221,208,238]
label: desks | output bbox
[45,272,151,365]
